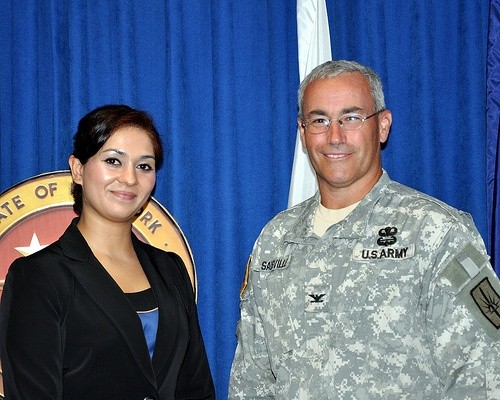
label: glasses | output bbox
[300,110,385,135]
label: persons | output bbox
[228,60,500,400]
[2,104,217,400]
